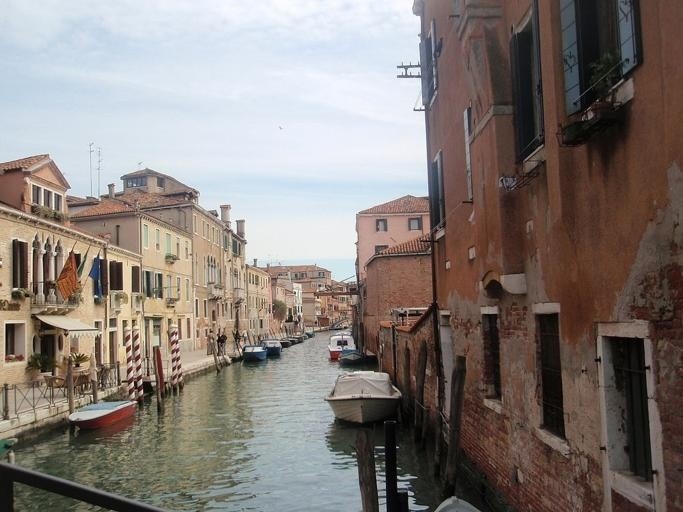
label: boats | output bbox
[330,322,352,330]
[241,331,316,362]
[323,369,402,425]
[327,334,357,360]
[67,399,137,428]
[70,415,136,449]
[338,349,362,363]
[433,494,481,512]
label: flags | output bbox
[57,252,78,301]
[89,254,102,298]
[77,255,88,282]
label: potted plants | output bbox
[70,353,90,367]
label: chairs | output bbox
[43,375,65,404]
[64,368,102,397]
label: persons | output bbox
[206,327,247,356]
